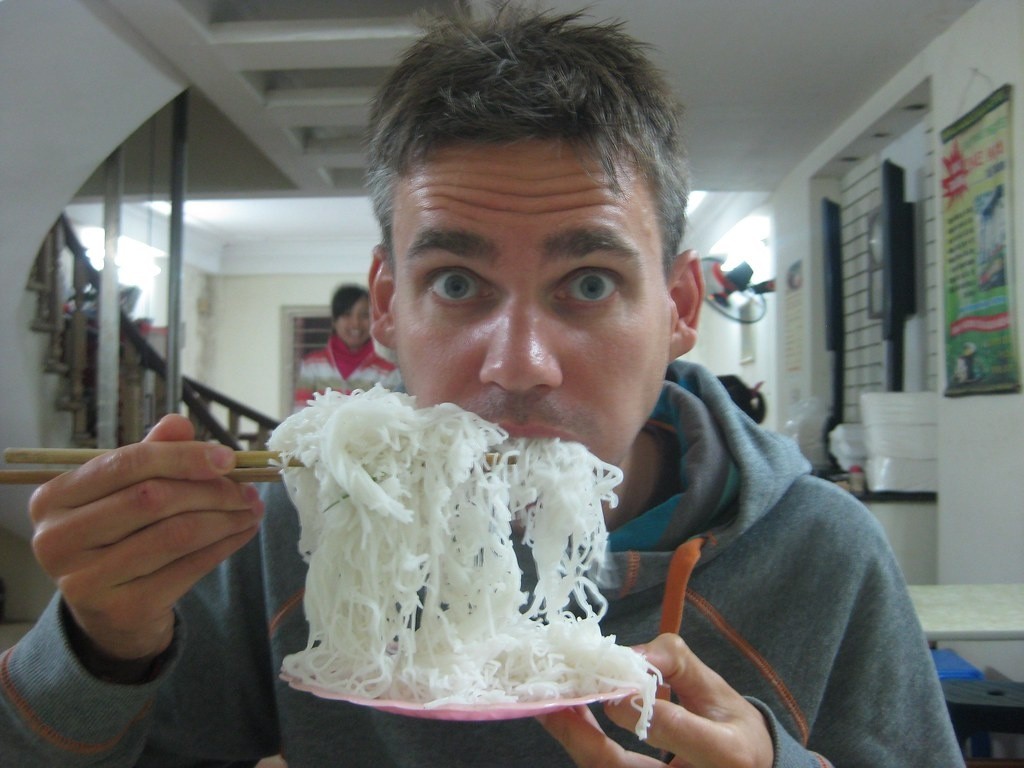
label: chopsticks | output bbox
[1,444,530,486]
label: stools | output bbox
[929,647,991,761]
[940,677,1024,768]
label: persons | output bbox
[293,283,397,411]
[1,21,963,768]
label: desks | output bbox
[902,584,1024,650]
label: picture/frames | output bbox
[866,203,885,318]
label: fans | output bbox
[699,256,778,324]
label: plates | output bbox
[276,655,653,719]
